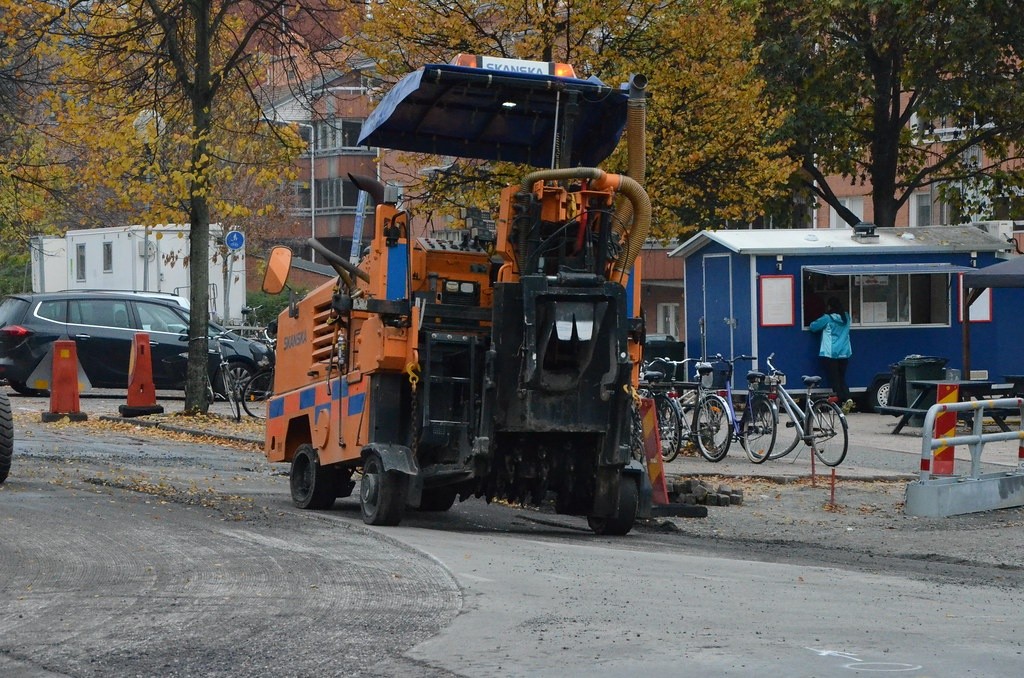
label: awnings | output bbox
[804,265,980,275]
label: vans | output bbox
[0,292,275,402]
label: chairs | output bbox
[115,310,128,328]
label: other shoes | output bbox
[829,410,838,415]
[842,399,853,415]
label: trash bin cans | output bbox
[898,354,949,427]
[645,340,686,402]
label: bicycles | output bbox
[241,304,262,338]
[242,326,277,419]
[178,330,240,421]
[632,352,849,467]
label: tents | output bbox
[962,255,1024,381]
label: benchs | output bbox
[991,406,1020,412]
[874,406,928,415]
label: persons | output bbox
[809,297,853,415]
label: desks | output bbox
[891,380,1012,434]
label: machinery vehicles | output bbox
[261,53,652,537]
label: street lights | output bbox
[133,110,166,291]
[243,116,315,263]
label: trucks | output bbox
[666,224,1024,413]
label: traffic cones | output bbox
[42,340,87,422]
[119,333,164,417]
